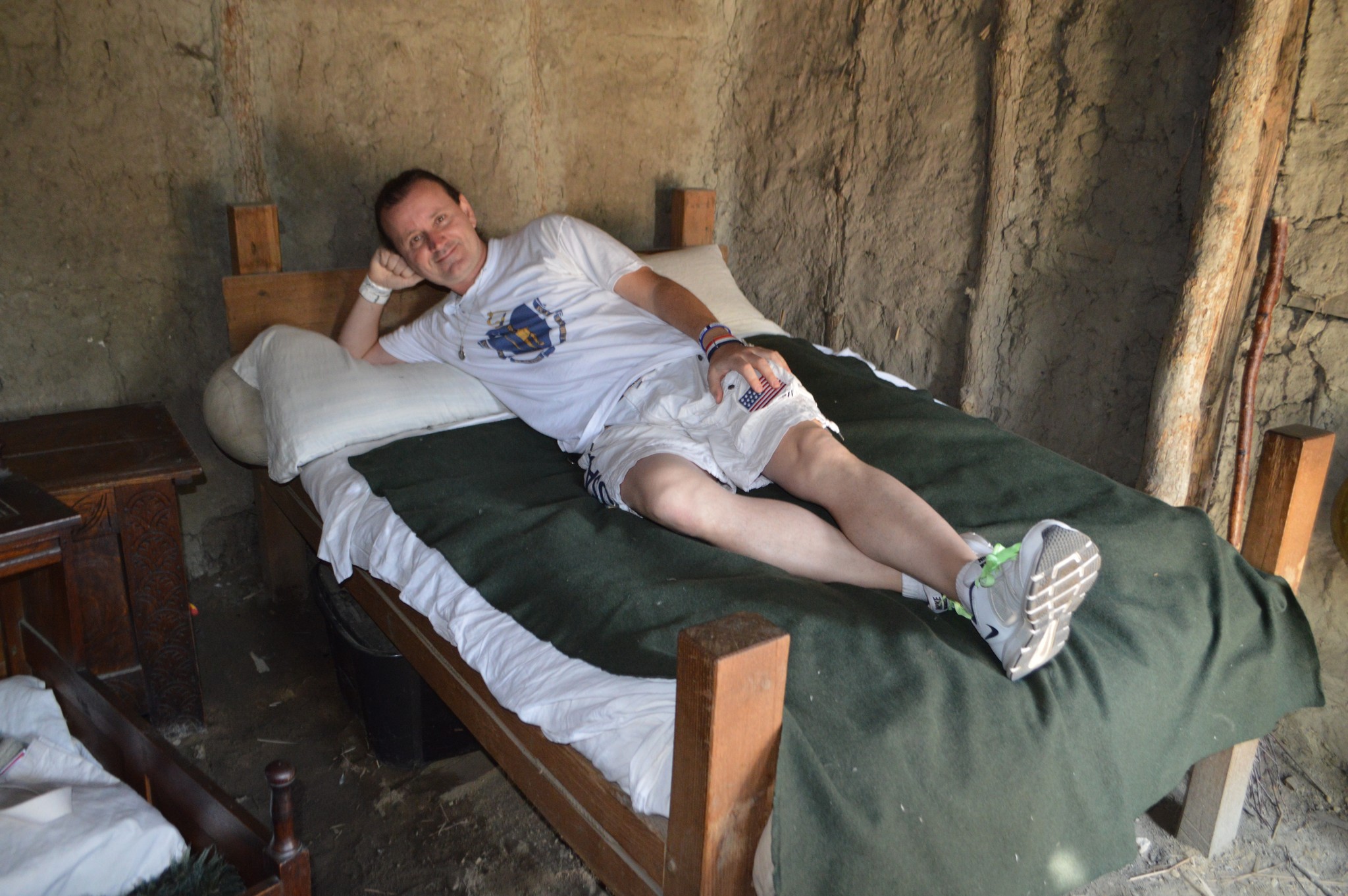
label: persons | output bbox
[339,167,1101,681]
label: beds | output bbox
[198,186,1338,896]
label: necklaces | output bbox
[454,272,482,360]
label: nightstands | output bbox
[0,405,204,722]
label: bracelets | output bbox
[699,323,732,351]
[359,274,393,305]
[706,334,745,362]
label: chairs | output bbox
[0,468,311,896]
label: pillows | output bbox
[233,325,512,482]
[643,242,792,339]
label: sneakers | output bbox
[962,518,1102,682]
[923,533,997,614]
